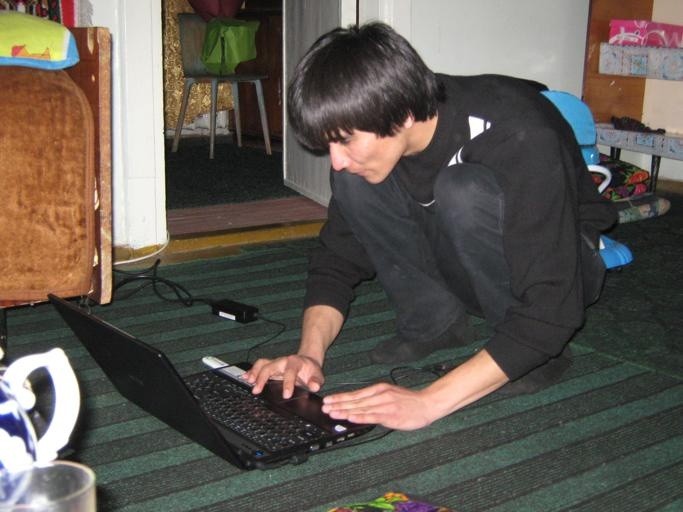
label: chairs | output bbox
[170,12,272,160]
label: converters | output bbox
[212,297,259,325]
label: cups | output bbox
[0,460,96,511]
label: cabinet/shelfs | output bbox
[229,9,282,144]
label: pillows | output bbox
[1,8,80,71]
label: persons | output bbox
[240,21,620,433]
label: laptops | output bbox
[47,293,377,470]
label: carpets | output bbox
[165,138,301,210]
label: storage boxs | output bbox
[608,20,683,47]
